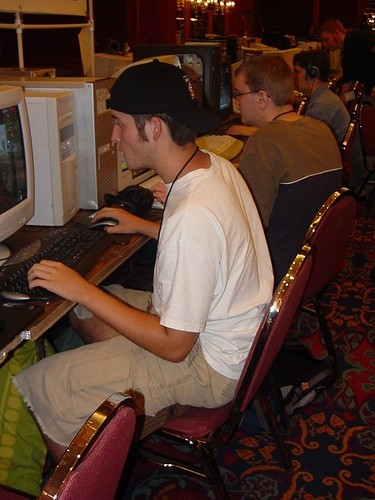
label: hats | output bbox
[106,59,222,133]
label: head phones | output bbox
[306,55,319,80]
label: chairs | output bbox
[339,114,366,195]
[131,244,314,500]
[0,392,136,500]
[266,188,358,417]
[358,101,375,184]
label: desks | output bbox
[0,120,250,363]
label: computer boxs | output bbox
[133,41,221,118]
[25,92,82,229]
[0,76,119,211]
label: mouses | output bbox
[91,220,117,230]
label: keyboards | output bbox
[0,226,111,302]
[195,135,244,161]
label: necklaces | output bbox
[273,110,297,120]
[146,146,200,312]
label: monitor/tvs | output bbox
[0,84,36,267]
[111,55,195,192]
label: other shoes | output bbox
[129,463,159,490]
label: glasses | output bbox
[232,90,271,101]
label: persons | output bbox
[292,51,351,141]
[14,58,274,463]
[359,15,369,32]
[318,19,375,201]
[234,54,343,421]
